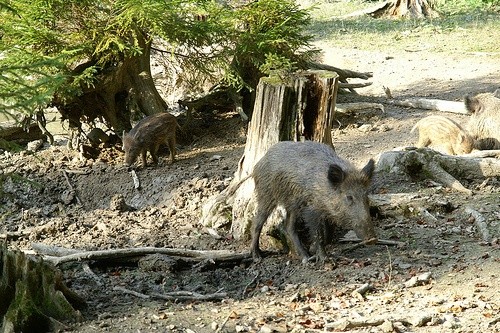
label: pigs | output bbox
[250,140,379,271]
[121,112,183,168]
[410,89,500,156]
